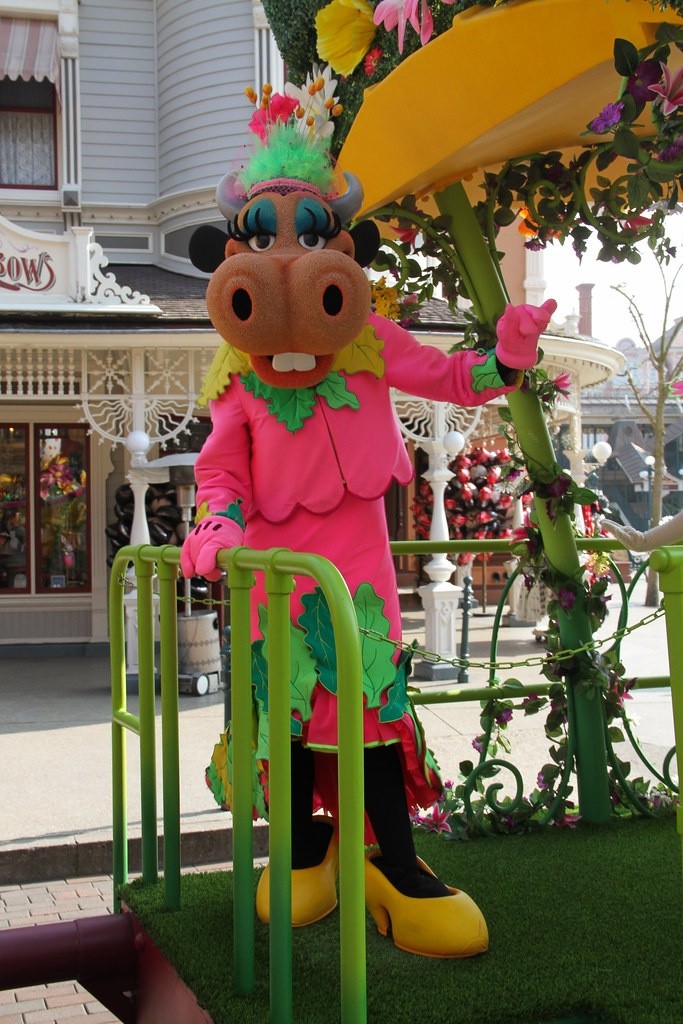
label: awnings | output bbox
[1,16,61,113]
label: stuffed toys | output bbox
[178,77,560,960]
[601,511,683,552]
